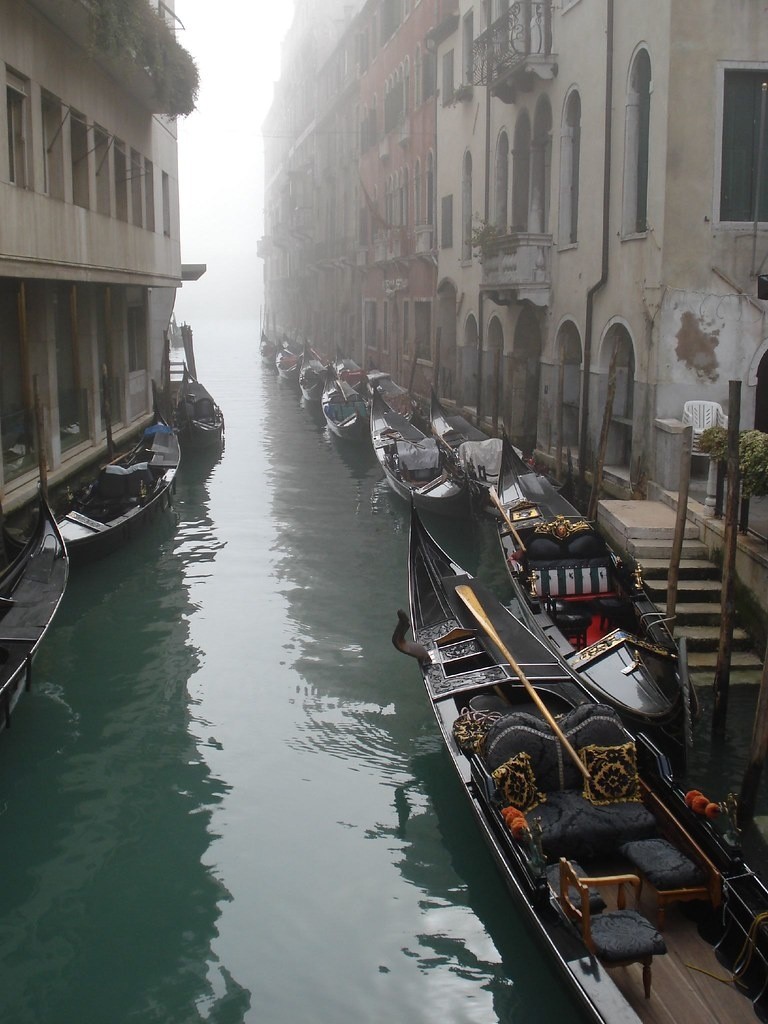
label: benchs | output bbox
[526,529,616,630]
[148,460,177,467]
[484,704,655,856]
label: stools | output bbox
[550,865,608,919]
[620,838,708,933]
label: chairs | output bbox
[546,595,587,651]
[559,856,667,999]
[681,400,729,456]
[101,462,153,499]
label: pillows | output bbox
[491,751,547,814]
[578,742,643,806]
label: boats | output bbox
[0,483,69,731]
[393,488,767,1022]
[370,380,490,516]
[429,384,573,519]
[489,424,704,767]
[259,329,416,442]
[57,397,182,558]
[176,361,224,447]
